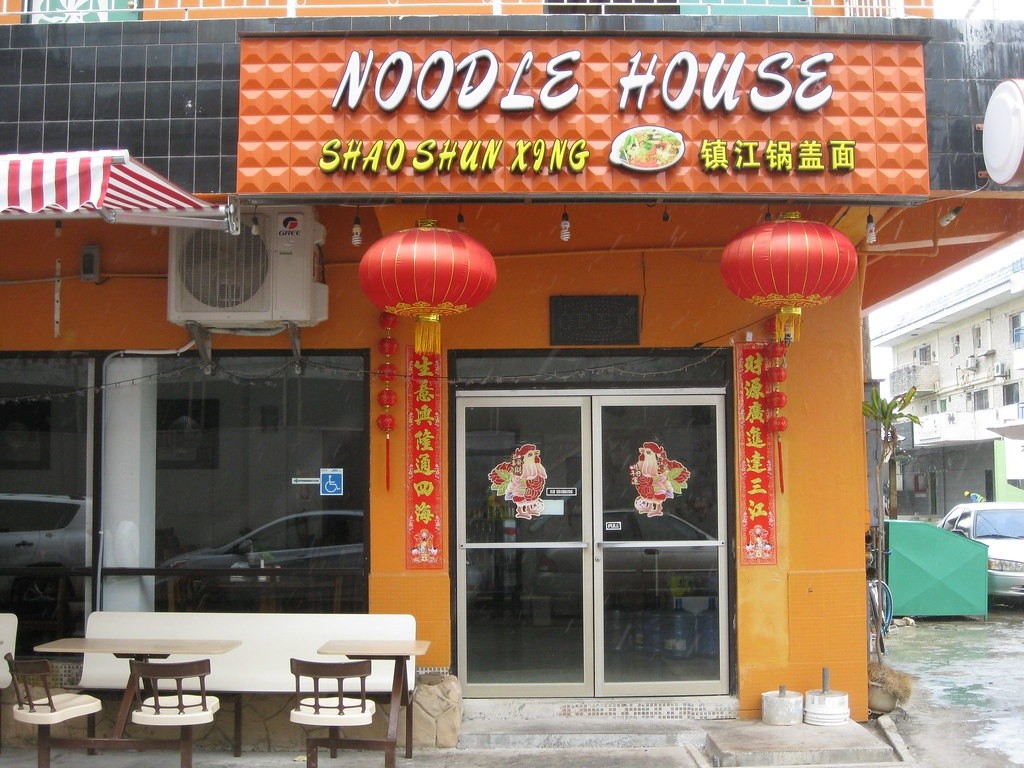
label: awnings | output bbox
[0,149,219,214]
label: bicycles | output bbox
[866,544,902,640]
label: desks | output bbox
[34,638,241,768]
[306,639,431,768]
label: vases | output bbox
[868,682,897,715]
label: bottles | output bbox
[696,598,719,657]
[664,597,695,659]
[605,596,665,655]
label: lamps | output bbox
[866,206,877,243]
[560,204,570,242]
[938,199,968,227]
[352,204,362,247]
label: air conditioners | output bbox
[966,357,977,368]
[168,204,328,329]
[995,363,1007,375]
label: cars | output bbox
[935,502,1024,599]
[156,511,485,623]
[520,506,718,620]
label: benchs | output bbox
[65,612,417,759]
[4,652,220,768]
[0,613,18,755]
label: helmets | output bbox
[964,491,969,497]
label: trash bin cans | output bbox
[883,519,991,622]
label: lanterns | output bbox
[376,312,398,493]
[359,218,497,355]
[763,317,788,494]
[721,211,858,343]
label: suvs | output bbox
[0,491,183,639]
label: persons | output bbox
[964,491,987,503]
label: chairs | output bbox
[291,659,376,727]
